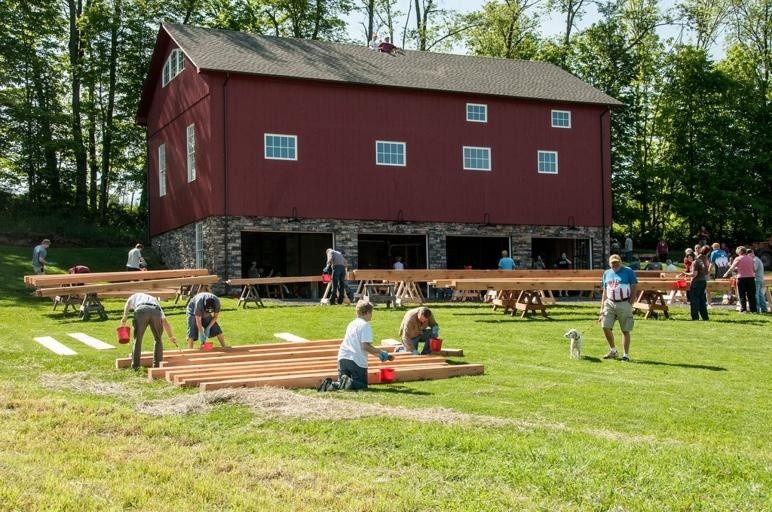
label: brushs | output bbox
[388,355,395,361]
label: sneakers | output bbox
[679,295,766,313]
[318,377,334,393]
[337,375,350,391]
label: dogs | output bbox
[563,329,582,360]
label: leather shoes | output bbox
[617,357,630,361]
[603,350,618,359]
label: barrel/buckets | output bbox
[117,326,130,344]
[429,338,444,352]
[677,274,687,289]
[380,368,396,383]
[321,272,331,283]
[730,278,736,288]
[201,342,213,349]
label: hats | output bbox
[747,248,753,254]
[609,255,622,264]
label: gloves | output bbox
[378,353,386,360]
[204,326,214,336]
[201,334,206,342]
[433,325,441,334]
[323,266,328,271]
[380,350,388,356]
[414,350,422,356]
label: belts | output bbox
[410,333,422,340]
[608,297,631,303]
[136,302,160,307]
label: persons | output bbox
[316,298,395,392]
[30,238,51,274]
[611,238,621,253]
[322,249,346,305]
[498,249,516,270]
[393,256,405,270]
[665,259,677,271]
[627,255,641,269]
[697,225,711,247]
[368,35,381,51]
[680,242,769,322]
[534,255,546,269]
[553,251,573,297]
[395,306,441,356]
[624,234,634,262]
[125,243,147,282]
[184,291,228,349]
[247,260,290,296]
[378,36,397,54]
[67,265,90,299]
[656,237,669,262]
[598,254,638,362]
[121,292,176,373]
